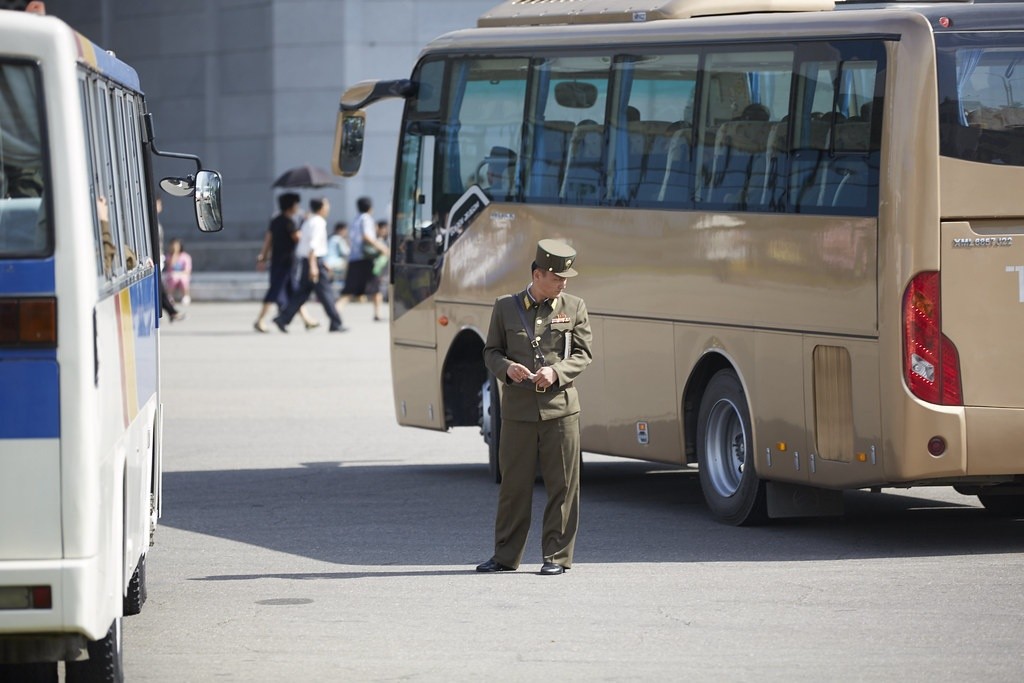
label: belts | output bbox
[510,380,554,393]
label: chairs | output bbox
[0,197,47,257]
[968,108,1024,130]
[502,120,871,208]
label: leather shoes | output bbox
[476,558,516,572]
[540,562,565,574]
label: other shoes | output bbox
[274,318,287,332]
[255,322,267,332]
[305,322,320,328]
[330,326,349,331]
[169,313,191,323]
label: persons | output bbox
[733,94,876,125]
[155,195,193,323]
[252,190,390,335]
[475,239,594,575]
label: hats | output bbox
[535,239,578,278]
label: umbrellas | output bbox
[269,164,339,208]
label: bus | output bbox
[0,9,223,682]
[330,1,1024,530]
